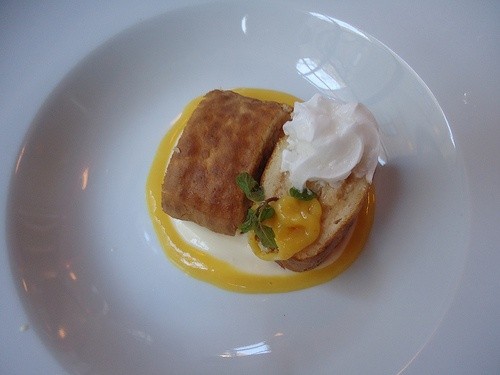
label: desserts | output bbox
[144,86,377,295]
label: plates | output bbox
[8,2,465,374]
